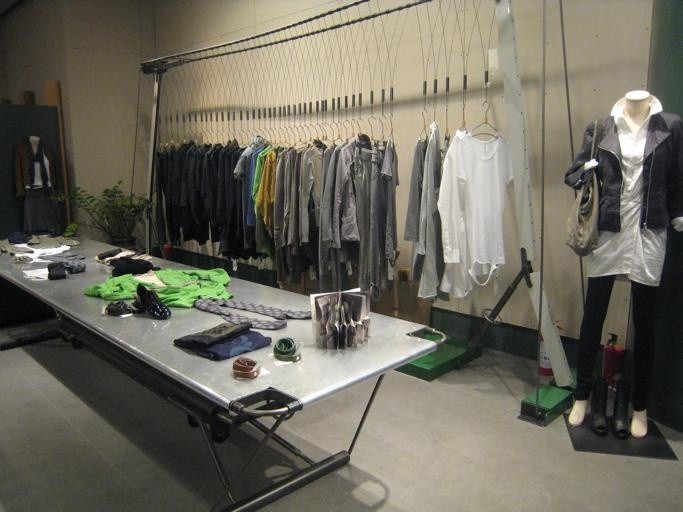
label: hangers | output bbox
[156,99,503,163]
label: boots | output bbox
[613,379,631,439]
[587,376,609,436]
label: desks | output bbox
[0,232,447,512]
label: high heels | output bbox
[136,283,171,320]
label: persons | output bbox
[564,90,683,438]
[15,136,61,234]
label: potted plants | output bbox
[47,180,155,252]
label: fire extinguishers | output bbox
[539,318,566,375]
[602,332,625,380]
[162,238,171,258]
